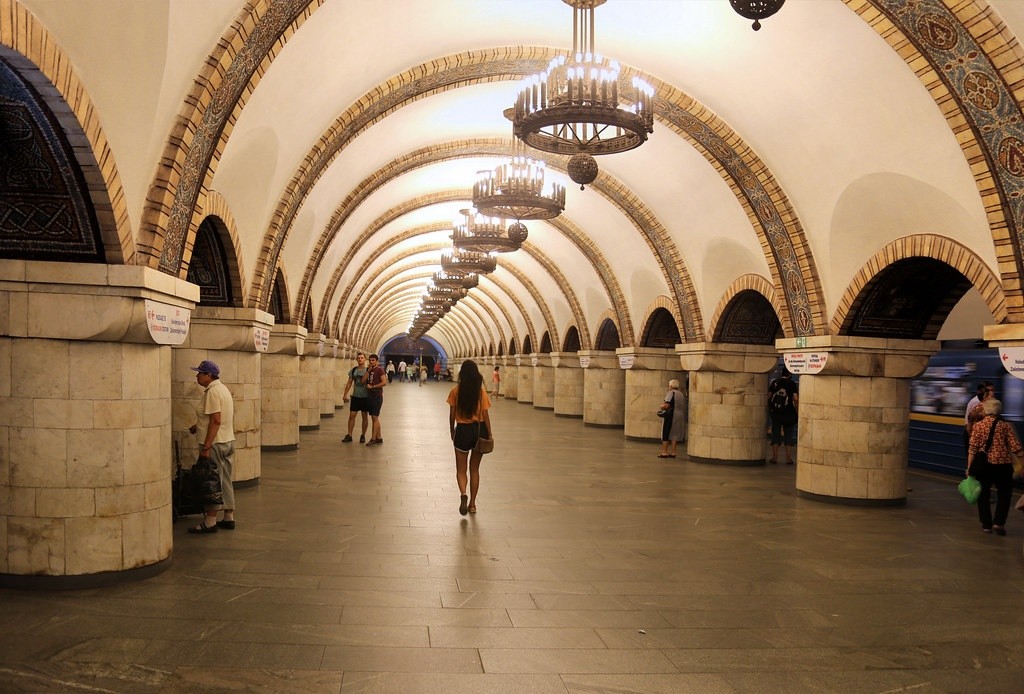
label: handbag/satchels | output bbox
[191,455,224,505]
[957,475,983,505]
[657,409,671,417]
[474,438,494,454]
[969,451,986,481]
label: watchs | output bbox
[202,446,210,450]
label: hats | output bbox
[190,361,220,377]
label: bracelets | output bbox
[372,385,375,388]
[450,425,455,432]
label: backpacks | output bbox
[768,380,794,422]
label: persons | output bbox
[768,366,799,464]
[657,379,684,458]
[397,359,407,382]
[385,360,395,384]
[405,362,428,384]
[446,360,493,515]
[188,360,237,534]
[489,366,501,400]
[342,352,387,446]
[964,382,1024,535]
[433,361,451,382]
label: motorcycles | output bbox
[439,368,452,382]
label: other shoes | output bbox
[983,529,992,535]
[467,505,476,513]
[360,435,365,443]
[342,434,352,442]
[459,493,468,516]
[188,522,218,533]
[786,459,794,465]
[658,453,669,458]
[669,454,676,458]
[992,524,1006,536]
[216,519,235,529]
[366,438,383,447]
[769,458,777,464]
